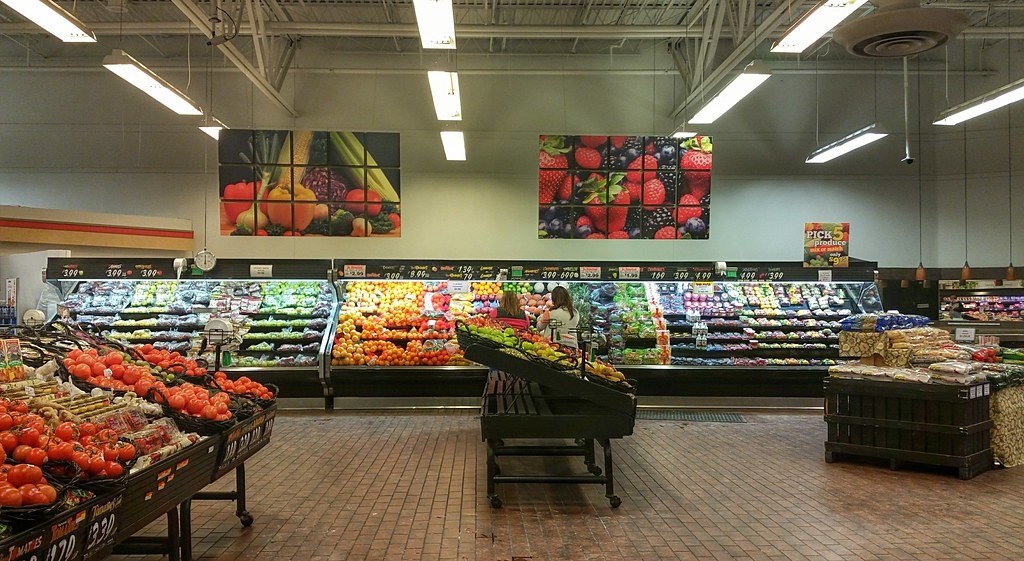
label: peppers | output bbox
[266,183,317,232]
[225,180,260,224]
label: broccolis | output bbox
[321,207,355,235]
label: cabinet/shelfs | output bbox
[0,259,1024,561]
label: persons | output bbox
[489,291,530,373]
[537,286,579,348]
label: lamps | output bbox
[916,55,926,281]
[770,0,869,53]
[198,45,230,140]
[413,0,457,50]
[0,0,98,42]
[932,46,1024,125]
[1006,40,1016,281]
[428,70,462,121]
[101,0,204,116]
[687,0,780,122]
[962,34,971,279]
[440,122,467,162]
[805,56,889,163]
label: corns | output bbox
[327,131,400,211]
[271,130,313,185]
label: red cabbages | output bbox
[302,168,349,212]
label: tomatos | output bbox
[346,186,381,217]
[0,344,274,506]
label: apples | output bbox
[476,295,495,313]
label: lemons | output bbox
[577,359,630,386]
[460,326,570,366]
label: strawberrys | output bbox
[539,136,713,239]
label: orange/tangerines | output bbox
[331,282,449,366]
